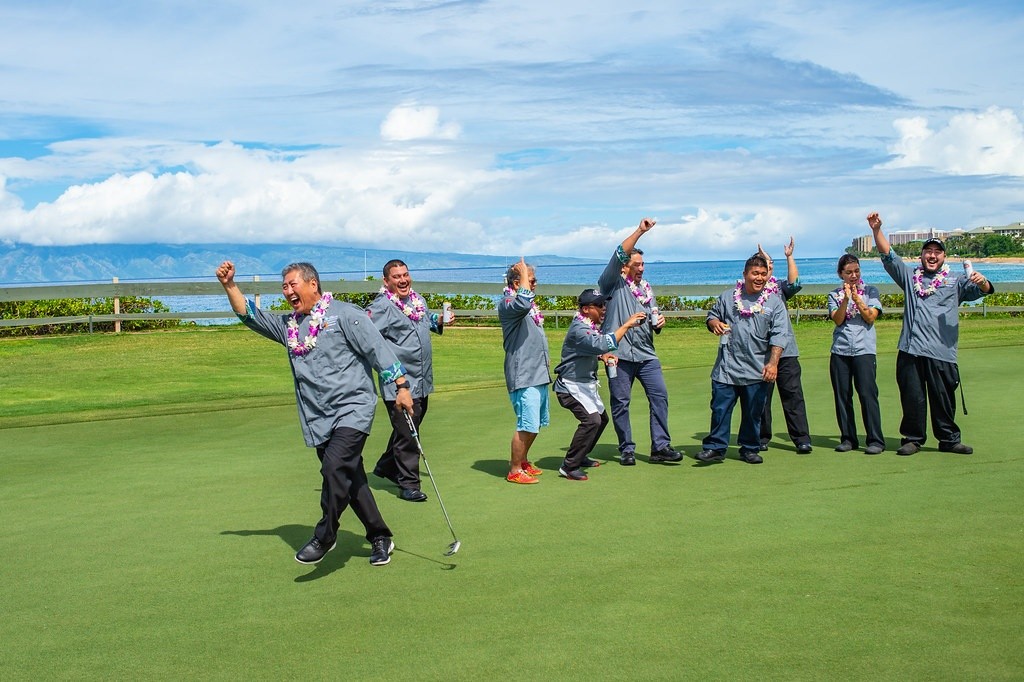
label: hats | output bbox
[578,288,613,308]
[922,237,946,252]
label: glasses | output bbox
[769,260,774,264]
[517,278,538,285]
[588,302,607,309]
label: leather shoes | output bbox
[695,447,727,461]
[620,453,636,465]
[761,443,768,451]
[648,443,683,462]
[797,441,813,453]
[400,489,428,501]
[739,448,763,463]
[372,465,400,485]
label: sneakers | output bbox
[897,442,918,455]
[939,441,973,454]
[835,439,857,452]
[559,466,588,480]
[864,446,885,454]
[579,459,599,467]
[520,460,542,475]
[506,470,539,483]
[369,537,395,565]
[294,536,337,564]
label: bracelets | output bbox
[396,381,411,389]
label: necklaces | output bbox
[763,275,778,294]
[837,277,864,319]
[573,312,602,335]
[913,264,950,296]
[288,292,333,354]
[380,284,425,321]
[620,273,653,305]
[733,280,768,317]
[502,286,544,327]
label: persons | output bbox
[827,254,886,454]
[597,218,683,466]
[752,236,813,452]
[364,259,455,502]
[867,211,995,455]
[498,256,553,483]
[552,288,646,480]
[215,261,415,564]
[694,255,788,463]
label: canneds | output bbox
[720,325,730,344]
[650,307,659,325]
[443,302,451,322]
[607,358,617,378]
[963,259,973,279]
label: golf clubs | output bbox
[402,406,460,556]
